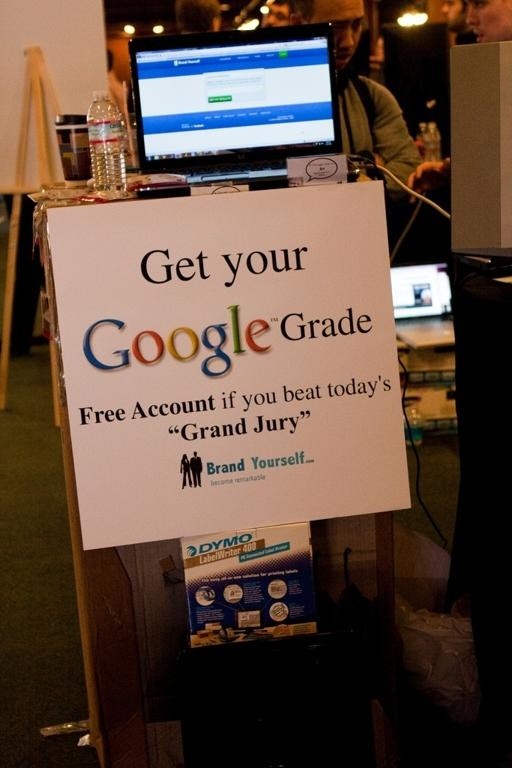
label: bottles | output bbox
[88,89,128,199]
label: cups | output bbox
[56,114,91,181]
[416,121,442,163]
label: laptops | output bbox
[390,259,456,350]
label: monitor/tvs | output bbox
[127,20,342,176]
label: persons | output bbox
[107,29,132,117]
[259,1,451,202]
[441,0,511,45]
